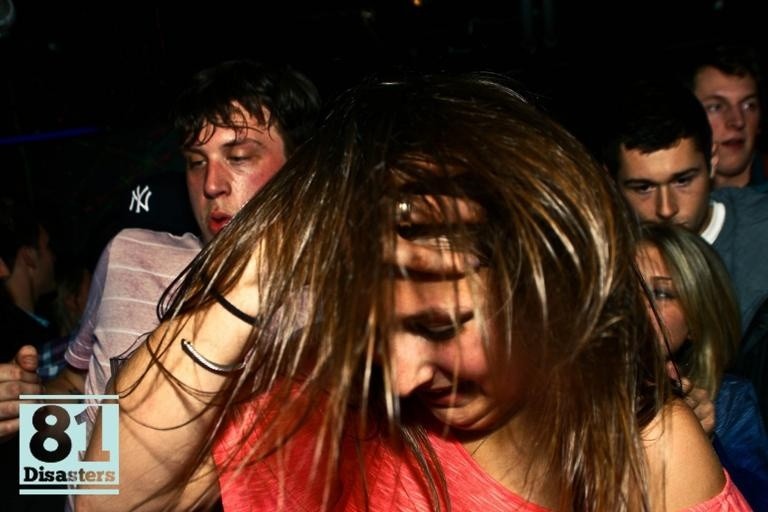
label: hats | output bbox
[122,183,174,231]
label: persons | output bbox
[1,48,767,512]
